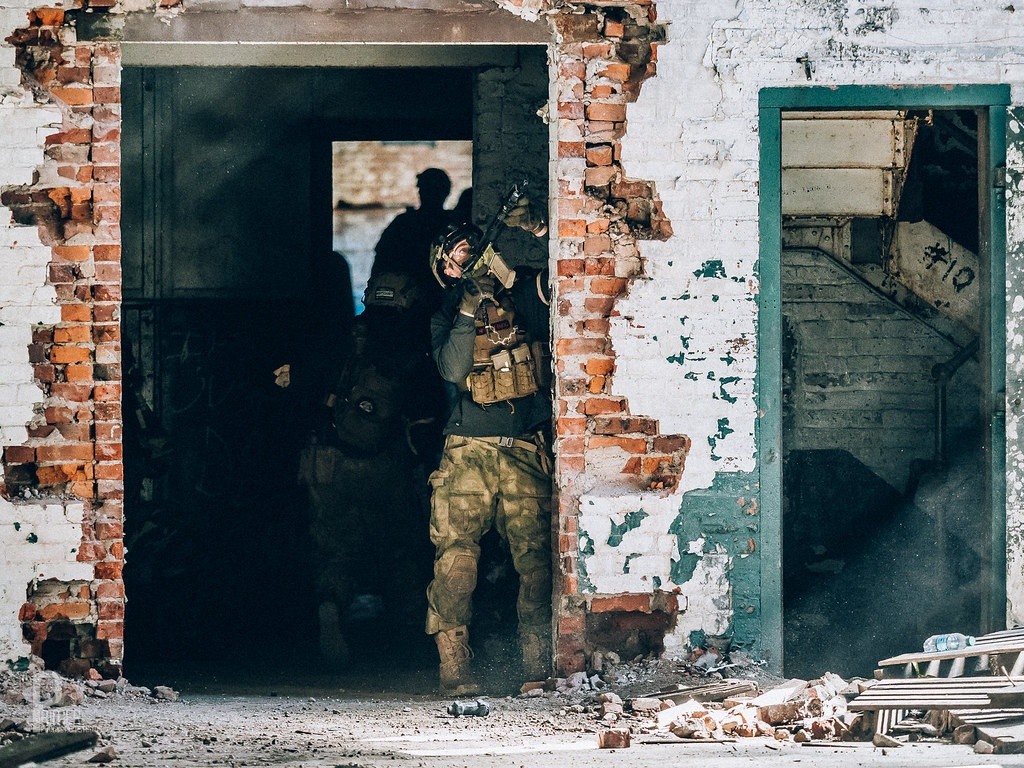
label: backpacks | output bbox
[336,339,431,453]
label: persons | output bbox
[297,169,472,677]
[424,189,553,697]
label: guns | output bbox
[458,179,532,290]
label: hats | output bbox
[363,271,422,315]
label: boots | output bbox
[518,634,554,689]
[433,625,480,697]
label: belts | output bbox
[472,436,553,476]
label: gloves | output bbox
[497,191,542,232]
[457,265,496,315]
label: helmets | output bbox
[453,187,473,215]
[429,219,484,268]
[416,168,452,196]
